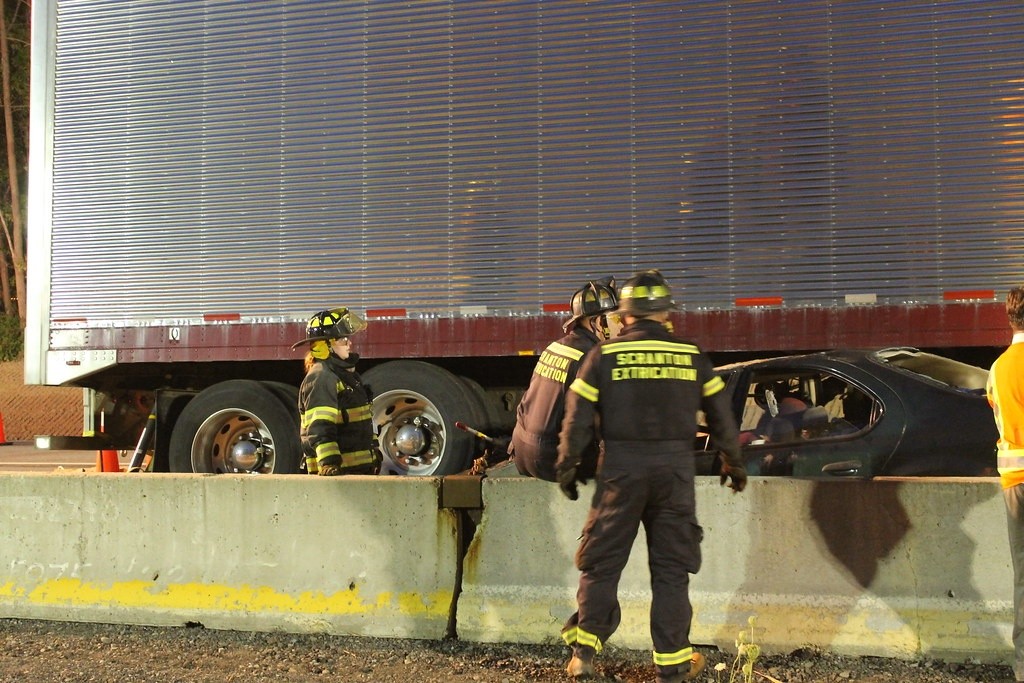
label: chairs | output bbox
[803,405,832,439]
[764,416,796,443]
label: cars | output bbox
[480,341,997,480]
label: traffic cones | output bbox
[89,409,123,474]
[0,412,12,445]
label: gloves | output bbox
[375,449,383,461]
[720,465,747,493]
[318,464,342,476]
[558,468,587,501]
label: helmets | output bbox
[609,274,675,316]
[563,281,618,327]
[291,311,352,348]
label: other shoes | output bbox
[1015,667,1024,682]
[656,653,705,683]
[567,657,595,676]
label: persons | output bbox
[553,272,747,682]
[296,310,384,474]
[504,282,625,483]
[984,286,1024,682]
[752,378,808,437]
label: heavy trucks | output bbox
[26,0,1024,475]
